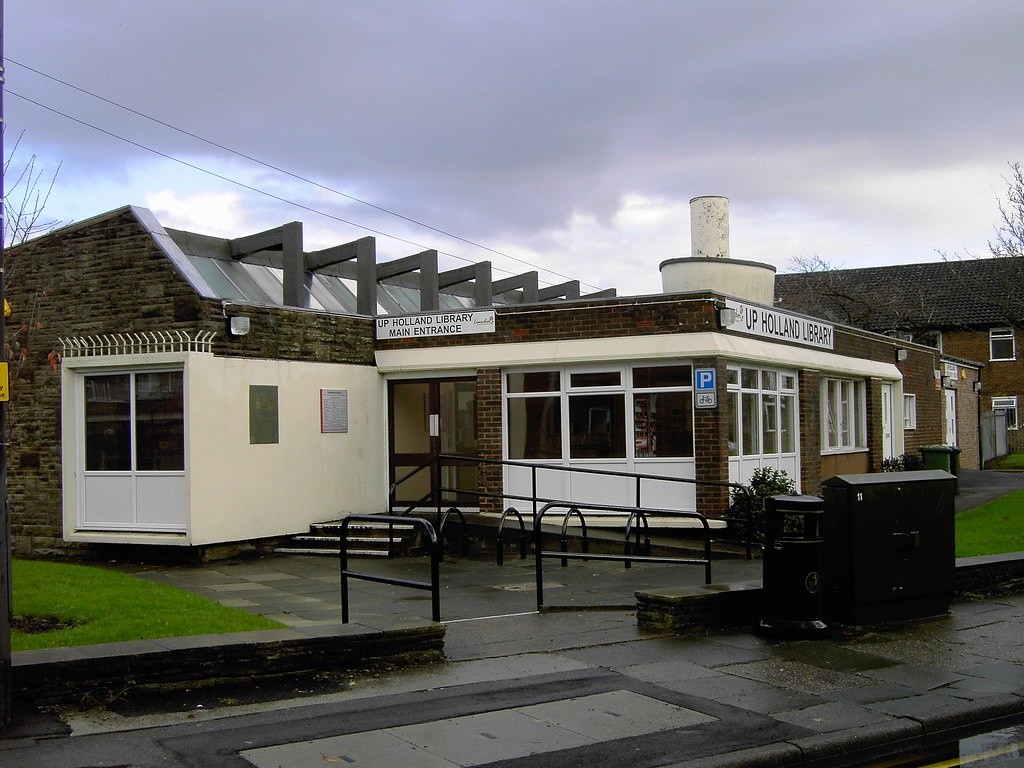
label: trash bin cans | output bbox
[822,469,960,633]
[916,443,962,497]
[760,495,831,637]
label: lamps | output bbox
[226,315,250,337]
[973,381,982,391]
[895,347,907,362]
